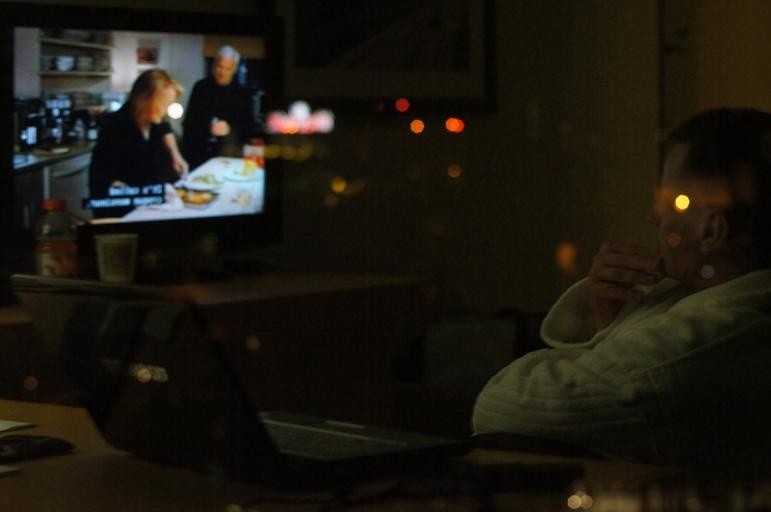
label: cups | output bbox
[93,232,139,282]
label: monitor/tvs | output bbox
[0,0,285,284]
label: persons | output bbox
[472,107,770,437]
[89,69,190,218]
[181,44,251,175]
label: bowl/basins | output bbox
[43,54,93,72]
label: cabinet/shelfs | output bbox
[108,31,204,135]
[0,400,771,511]
[14,28,114,113]
[14,138,92,218]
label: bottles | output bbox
[35,200,79,281]
[45,107,100,146]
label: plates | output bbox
[183,179,221,192]
[225,169,260,182]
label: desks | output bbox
[83,153,270,225]
[0,265,445,474]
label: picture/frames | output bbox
[263,0,498,122]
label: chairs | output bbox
[466,305,611,460]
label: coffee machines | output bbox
[14,97,45,152]
